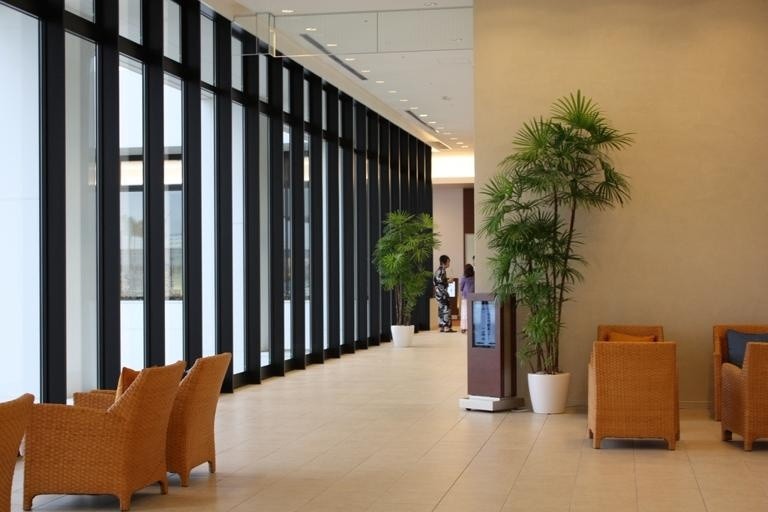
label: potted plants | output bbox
[372,210,442,347]
[474,90,637,413]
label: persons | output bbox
[456,264,477,333]
[433,254,458,333]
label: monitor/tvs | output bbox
[447,280,456,298]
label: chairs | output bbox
[587,325,680,450]
[0,352,231,512]
[711,324,768,452]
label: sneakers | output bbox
[440,329,457,333]
